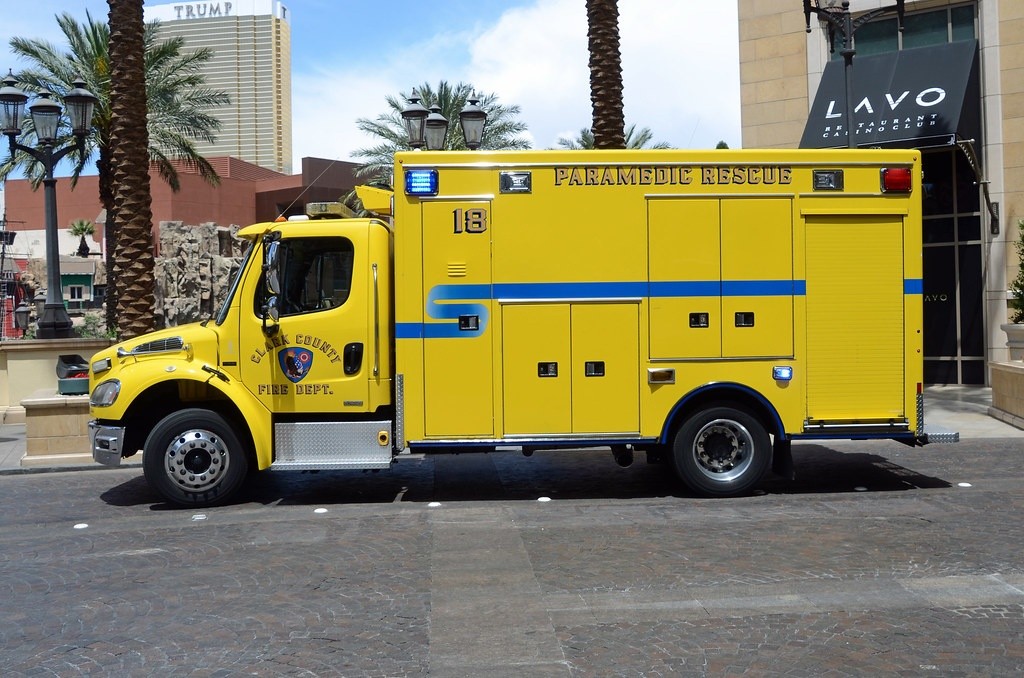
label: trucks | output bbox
[87,149,959,508]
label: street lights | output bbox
[0,67,100,338]
[0,209,25,281]
